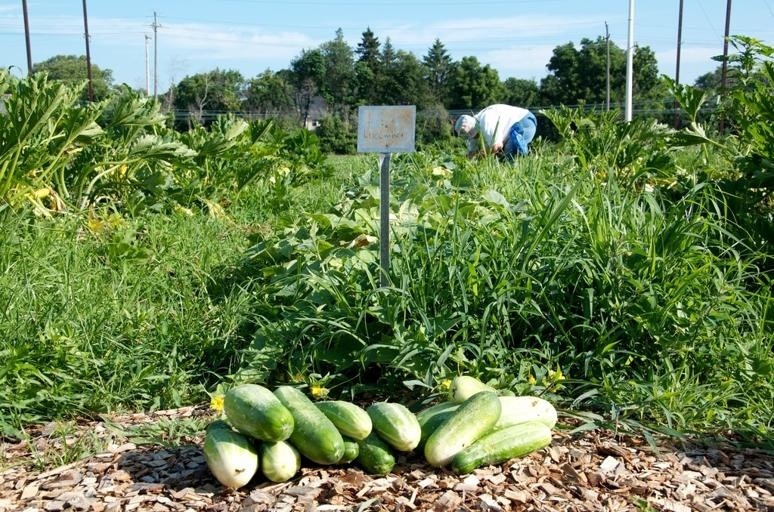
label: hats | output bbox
[455,115,480,140]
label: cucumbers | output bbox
[202,371,558,489]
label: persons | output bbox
[455,104,537,162]
